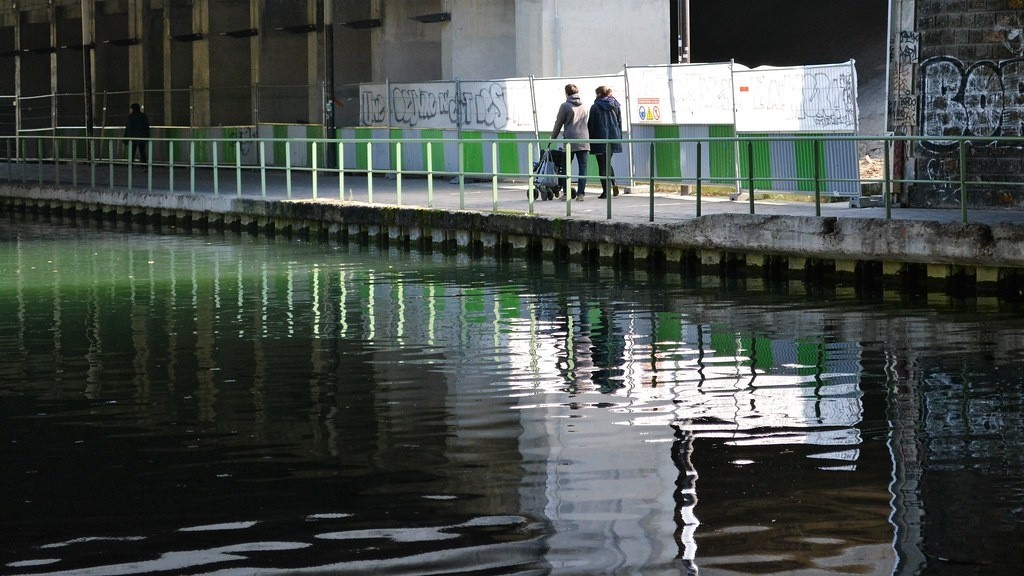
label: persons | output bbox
[550,84,591,201]
[122,103,150,174]
[587,85,625,198]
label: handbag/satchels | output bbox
[537,146,558,187]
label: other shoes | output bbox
[140,168,146,174]
[598,191,607,199]
[558,195,567,199]
[576,194,584,202]
[613,186,619,196]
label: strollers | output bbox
[524,141,579,202]
